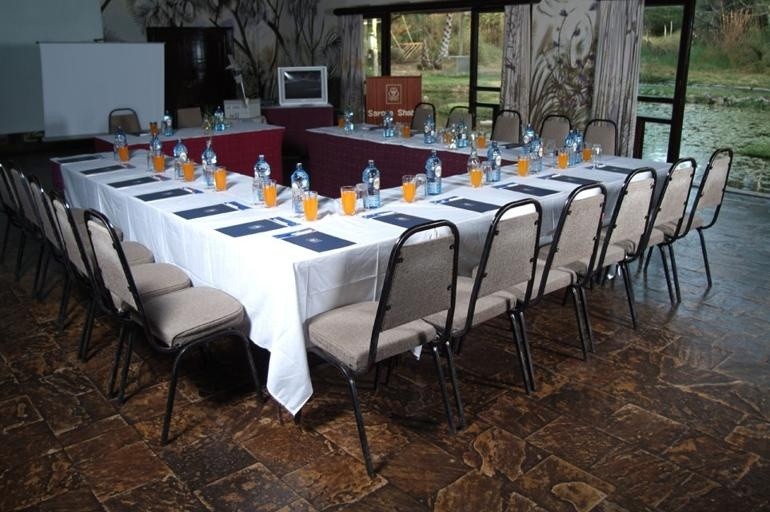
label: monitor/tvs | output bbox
[277,66,328,106]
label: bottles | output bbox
[113,106,601,217]
